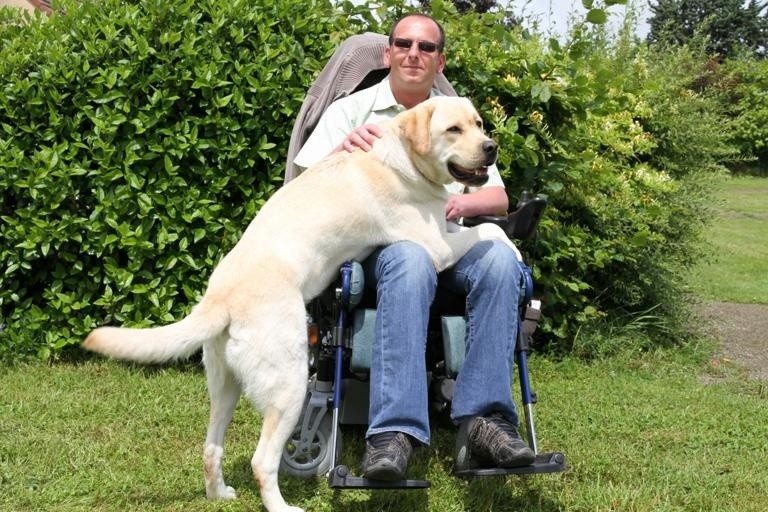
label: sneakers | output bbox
[362,431,415,481]
[467,410,535,468]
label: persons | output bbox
[292,13,539,485]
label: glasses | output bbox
[393,38,439,52]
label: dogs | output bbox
[79,94,525,512]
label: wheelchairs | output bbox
[279,28,570,490]
